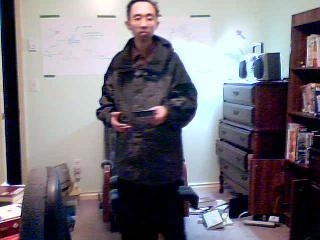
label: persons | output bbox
[96,0,197,240]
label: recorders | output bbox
[239,42,281,81]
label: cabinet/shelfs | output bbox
[248,7,320,240]
[215,80,287,221]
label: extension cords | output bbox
[242,220,275,227]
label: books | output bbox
[0,182,26,225]
[304,34,320,68]
[285,122,320,166]
[299,81,320,118]
[189,196,233,231]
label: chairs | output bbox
[19,165,71,240]
[101,123,189,222]
